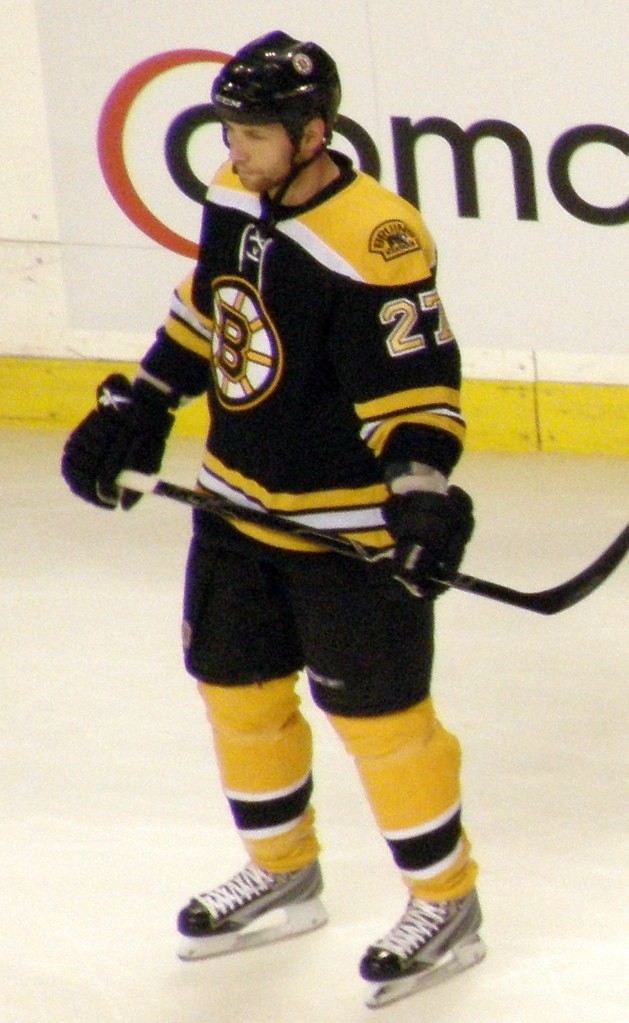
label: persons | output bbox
[58,29,487,1008]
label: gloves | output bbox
[374,485,475,604]
[62,372,179,511]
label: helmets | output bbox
[212,31,340,126]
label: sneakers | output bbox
[174,864,332,959]
[358,892,489,1011]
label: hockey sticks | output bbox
[115,467,628,617]
